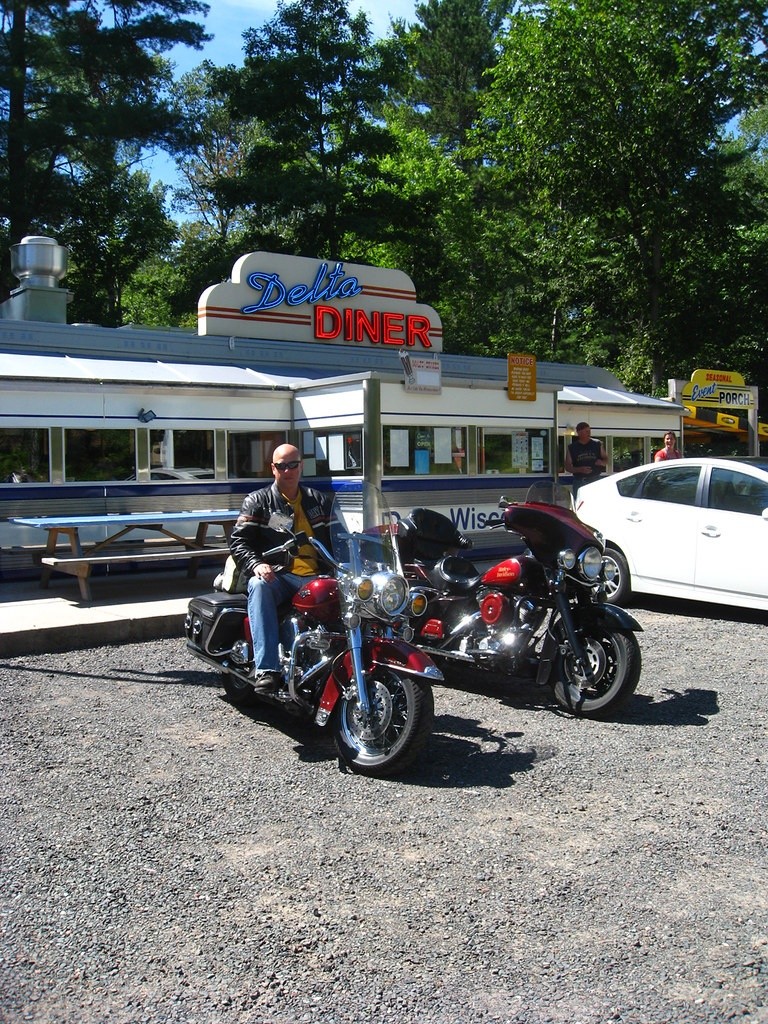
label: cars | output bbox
[574,454,767,613]
[123,463,239,481]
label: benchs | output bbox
[0,534,235,603]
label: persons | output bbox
[654,431,682,462]
[566,422,609,501]
[230,444,351,692]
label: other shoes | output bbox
[254,672,281,691]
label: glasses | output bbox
[273,461,301,470]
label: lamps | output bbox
[138,408,157,423]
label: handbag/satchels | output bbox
[221,555,253,594]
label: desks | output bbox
[6,507,241,603]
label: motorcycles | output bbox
[358,480,645,720]
[185,480,447,778]
